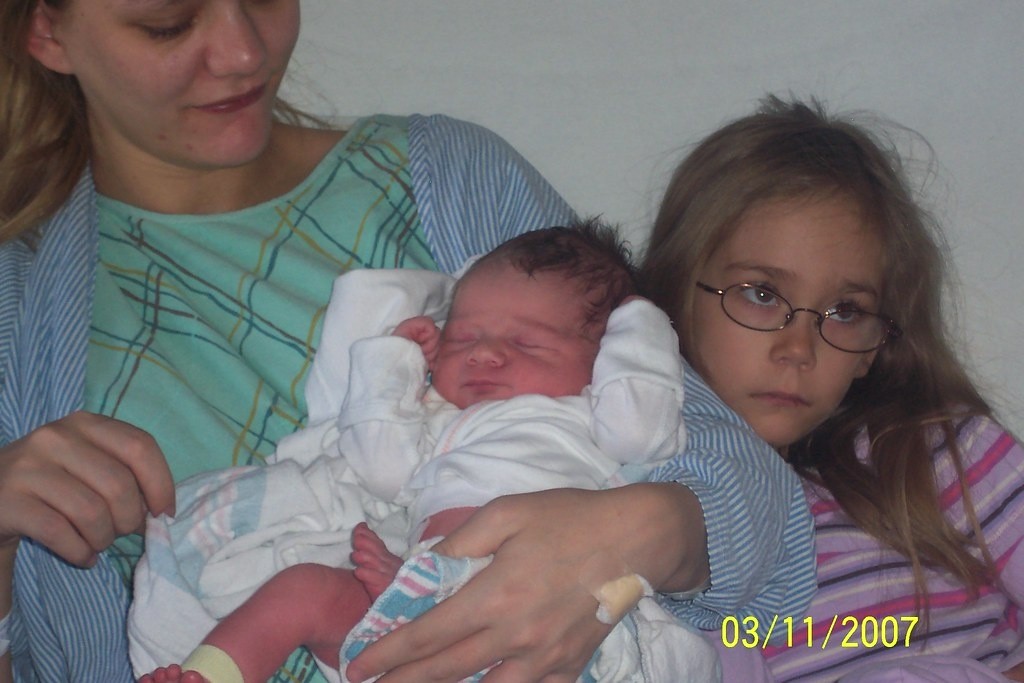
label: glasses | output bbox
[695,280,903,353]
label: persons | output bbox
[134,225,686,683]
[0,1,818,683]
[644,102,1024,683]
